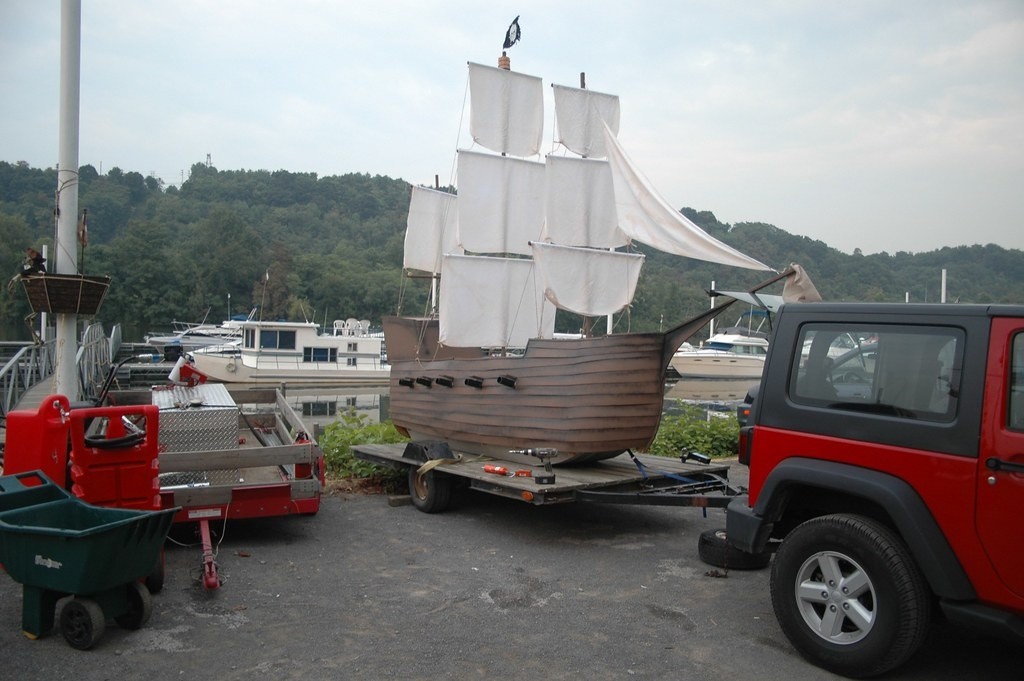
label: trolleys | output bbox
[0,469,183,649]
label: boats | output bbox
[181,269,392,389]
[143,292,259,355]
[667,303,775,376]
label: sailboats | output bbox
[380,16,798,464]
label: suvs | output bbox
[727,303,1023,678]
